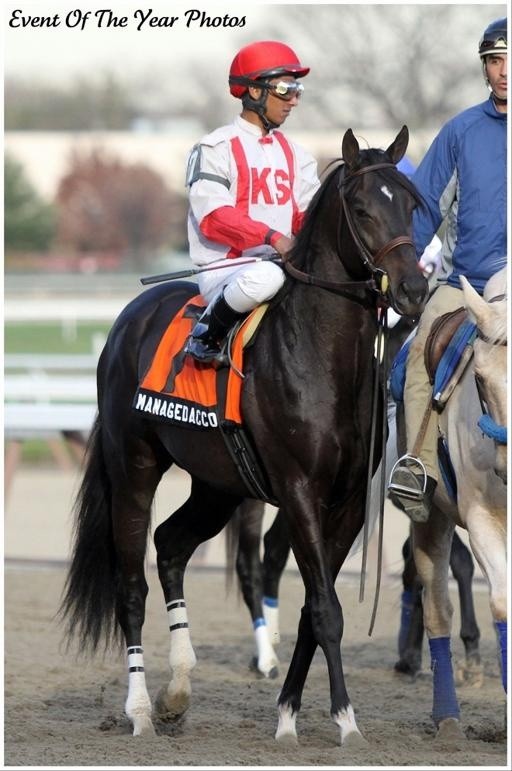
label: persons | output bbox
[387,15,507,523]
[180,42,321,363]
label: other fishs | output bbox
[57,122,507,766]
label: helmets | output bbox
[229,42,310,98]
[478,18,507,57]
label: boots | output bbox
[391,466,437,524]
[188,284,248,364]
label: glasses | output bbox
[230,74,304,102]
